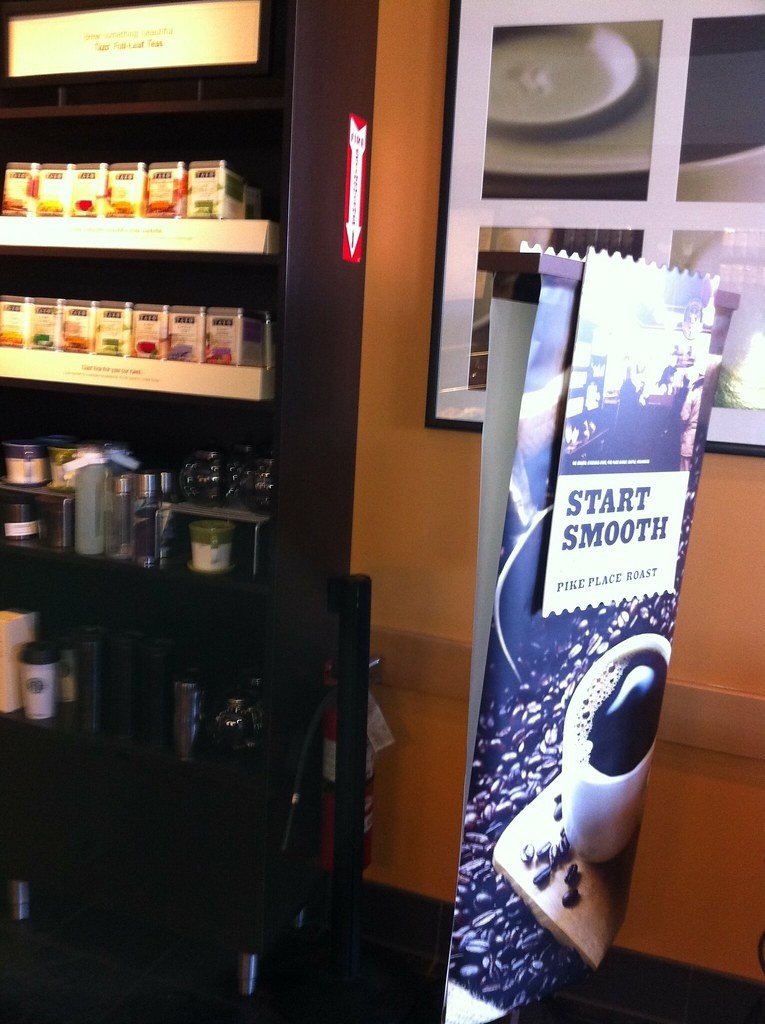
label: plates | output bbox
[487,25,639,130]
[186,559,235,575]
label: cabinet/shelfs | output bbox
[0,0,383,1024]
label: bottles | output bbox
[104,471,175,571]
[73,449,109,556]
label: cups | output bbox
[57,645,81,703]
[0,495,72,551]
[177,441,283,517]
[0,434,77,492]
[188,520,237,569]
[173,678,200,762]
[18,641,62,720]
[560,632,672,863]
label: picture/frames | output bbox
[423,0,765,456]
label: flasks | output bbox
[106,632,169,736]
